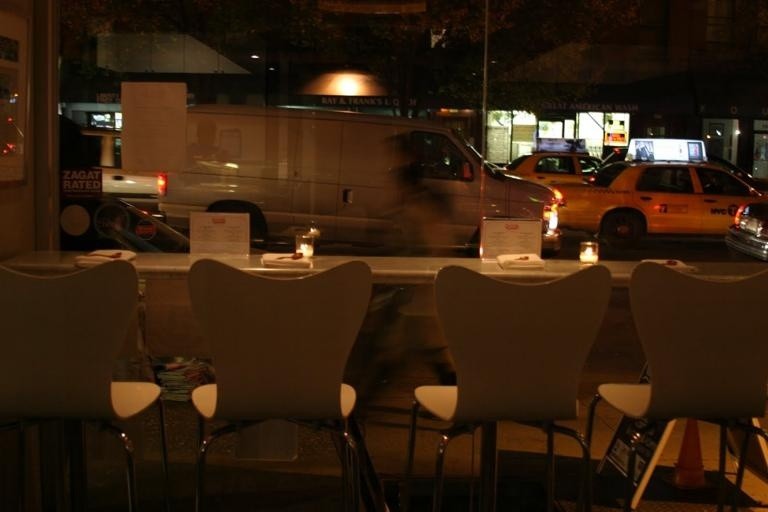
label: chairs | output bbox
[583,261,767,512]
[402,265,611,512]
[0,260,170,512]
[188,258,361,512]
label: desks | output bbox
[0,249,768,511]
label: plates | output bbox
[641,259,696,272]
[88,249,137,262]
[261,251,314,270]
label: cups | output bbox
[579,241,599,264]
[295,231,315,257]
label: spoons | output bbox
[276,253,303,260]
[89,252,122,258]
[660,261,677,265]
